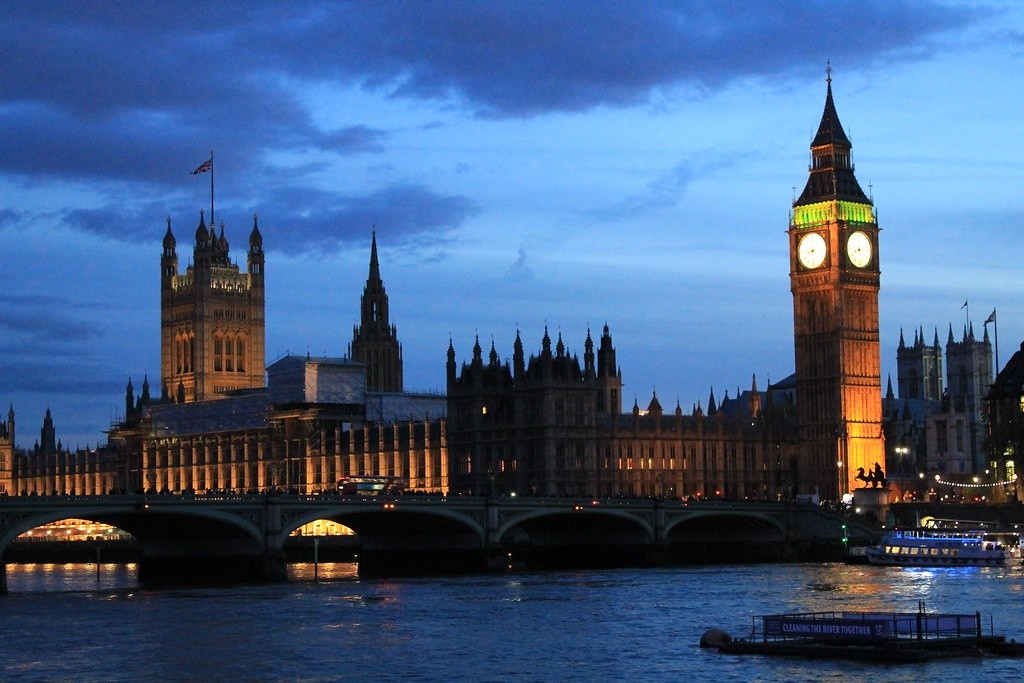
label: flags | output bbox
[984,311,995,325]
[191,158,212,174]
[961,301,967,308]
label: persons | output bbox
[21,489,46,496]
[5,489,8,496]
[868,469,874,477]
[954,550,957,555]
[206,486,282,500]
[986,543,1008,552]
[378,488,443,496]
[146,487,195,500]
[311,489,339,500]
[1010,544,1019,559]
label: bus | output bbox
[337,476,405,496]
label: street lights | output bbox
[919,473,923,503]
[326,521,330,535]
[47,530,51,542]
[837,461,842,509]
[716,492,720,503]
[696,493,701,504]
[67,530,71,541]
[27,530,32,542]
[1012,474,1017,503]
[895,448,908,503]
[935,474,940,502]
[87,530,91,536]
[973,476,978,501]
[108,528,113,540]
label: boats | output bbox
[718,603,1024,660]
[895,518,1020,546]
[865,532,1009,567]
